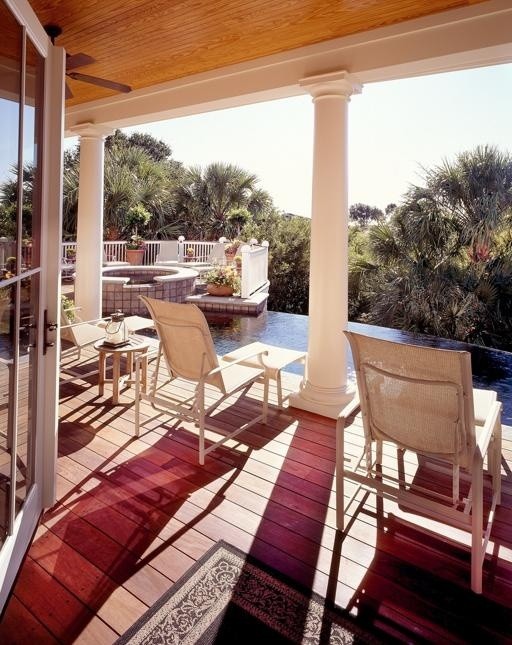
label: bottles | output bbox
[107,312,128,344]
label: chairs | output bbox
[338,328,501,597]
[134,293,310,465]
[60,307,157,384]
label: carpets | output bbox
[113,538,385,645]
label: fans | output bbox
[43,23,136,101]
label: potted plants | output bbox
[205,241,244,296]
[126,233,148,265]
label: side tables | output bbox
[93,333,150,406]
